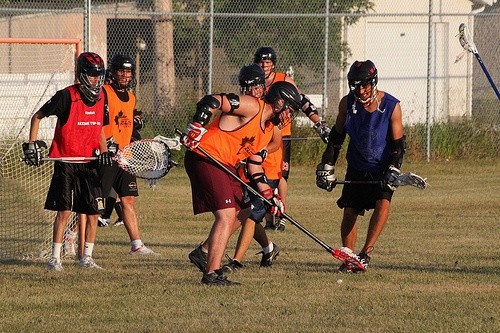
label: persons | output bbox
[22,51,113,273]
[97,68,125,229]
[316,61,406,273]
[252,46,331,232]
[60,54,161,259]
[221,63,284,274]
[183,80,301,289]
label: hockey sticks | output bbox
[175,126,368,272]
[335,171,428,191]
[282,136,320,140]
[458,22,500,102]
[20,138,171,181]
[153,135,182,152]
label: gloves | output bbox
[263,188,285,216]
[314,163,337,193]
[133,114,145,132]
[20,140,47,167]
[178,121,207,150]
[100,151,115,168]
[381,165,398,191]
[312,120,333,144]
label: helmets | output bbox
[264,81,302,130]
[345,60,379,107]
[238,63,266,98]
[75,51,105,107]
[109,54,138,92]
[253,45,277,66]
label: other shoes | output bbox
[112,219,125,228]
[337,246,374,276]
[263,217,287,231]
[96,216,111,227]
[47,257,64,272]
[80,256,105,270]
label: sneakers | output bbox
[187,241,210,274]
[255,243,281,267]
[61,233,76,258]
[222,254,245,275]
[129,245,159,256]
[202,268,242,285]
